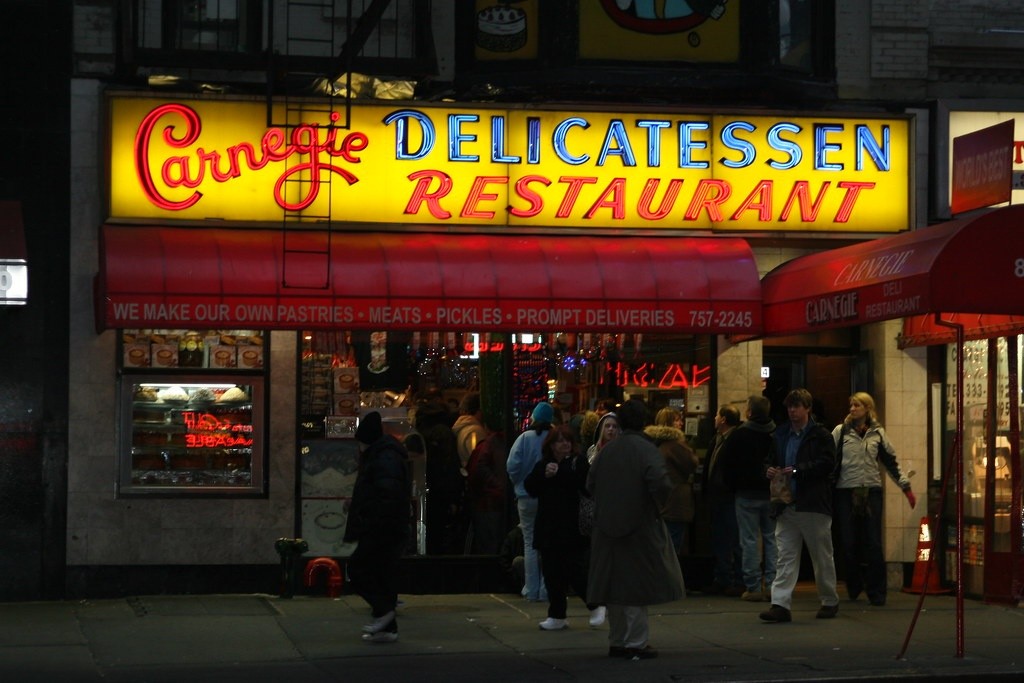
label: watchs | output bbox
[791,464,798,475]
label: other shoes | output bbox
[361,631,398,642]
[609,645,625,657]
[867,585,884,606]
[743,591,762,601]
[816,604,839,618]
[846,570,862,600]
[765,590,772,599]
[759,605,791,622]
[624,646,657,660]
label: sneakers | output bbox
[538,617,569,630]
[588,605,606,627]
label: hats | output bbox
[532,402,553,423]
[354,411,383,444]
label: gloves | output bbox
[902,487,915,509]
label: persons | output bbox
[583,401,685,661]
[341,410,428,644]
[451,391,919,629]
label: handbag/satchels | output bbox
[770,467,792,504]
[577,496,596,538]
[828,429,846,488]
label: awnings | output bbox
[90,217,765,337]
[762,202,1023,339]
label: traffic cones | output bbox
[900,517,950,595]
